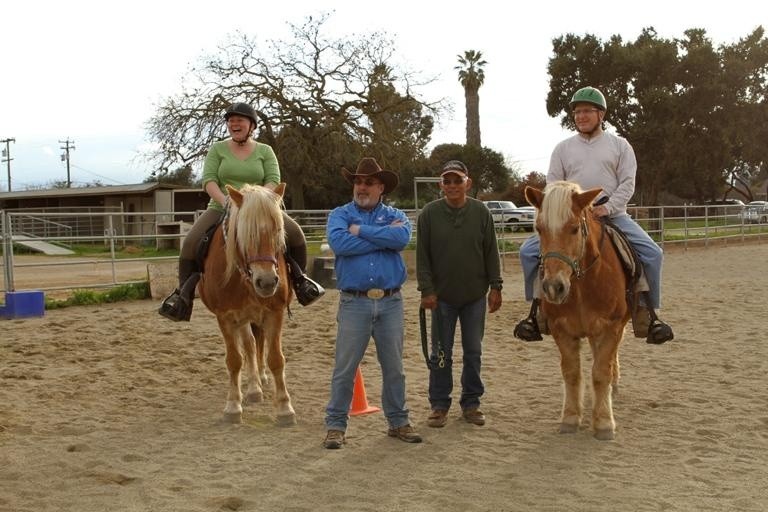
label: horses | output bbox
[198,183,297,427]
[524,180,638,439]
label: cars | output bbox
[482,200,536,232]
[738,200,768,224]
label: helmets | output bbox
[222,102,259,127]
[567,85,610,110]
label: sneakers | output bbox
[426,408,449,428]
[645,319,675,346]
[461,408,486,427]
[323,429,346,449]
[512,319,544,343]
[387,424,422,444]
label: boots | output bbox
[290,243,325,305]
[158,258,194,324]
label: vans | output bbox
[705,198,745,216]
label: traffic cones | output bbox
[347,363,381,419]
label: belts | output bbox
[340,286,401,301]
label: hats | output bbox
[439,160,469,179]
[340,157,399,197]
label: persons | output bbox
[414,158,504,429]
[320,156,423,450]
[158,102,325,323]
[514,86,674,347]
